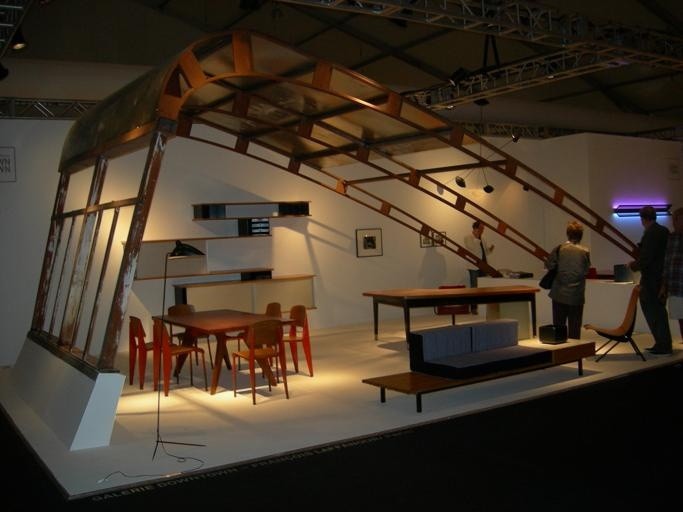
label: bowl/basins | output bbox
[540,324,567,345]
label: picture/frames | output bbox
[355,227,385,258]
[420,232,433,249]
[432,230,448,245]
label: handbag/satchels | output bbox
[539,243,563,289]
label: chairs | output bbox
[583,283,650,364]
[261,301,283,379]
[231,318,290,405]
[126,315,178,391]
[167,302,214,378]
[282,305,316,377]
[149,315,209,398]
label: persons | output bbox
[544,223,591,339]
[462,221,495,315]
[630,206,674,354]
[657,208,683,353]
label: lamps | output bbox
[436,96,532,198]
[150,239,207,464]
[610,200,674,223]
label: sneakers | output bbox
[644,344,657,352]
[648,350,672,356]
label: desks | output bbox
[349,317,597,415]
[147,307,298,396]
[361,283,542,344]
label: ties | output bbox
[479,238,486,263]
